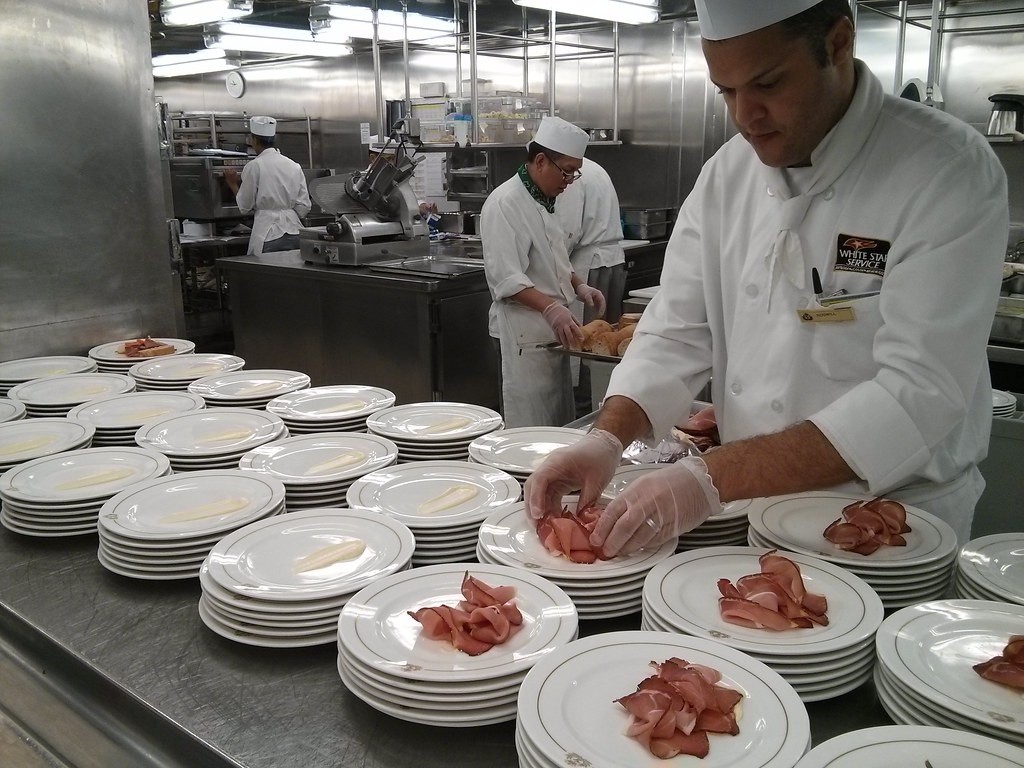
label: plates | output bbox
[0,333,1024,768]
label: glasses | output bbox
[534,150,582,182]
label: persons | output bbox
[361,134,438,215]
[524,0,1009,558]
[558,157,628,413]
[223,116,312,257]
[479,116,606,429]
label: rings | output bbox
[646,518,659,533]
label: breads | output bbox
[565,312,642,357]
[140,344,175,357]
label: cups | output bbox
[454,113,472,147]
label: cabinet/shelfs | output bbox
[217,250,497,418]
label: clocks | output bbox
[226,72,244,98]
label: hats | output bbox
[533,116,590,159]
[249,116,277,136]
[692,0,825,41]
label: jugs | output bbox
[986,94,1024,135]
